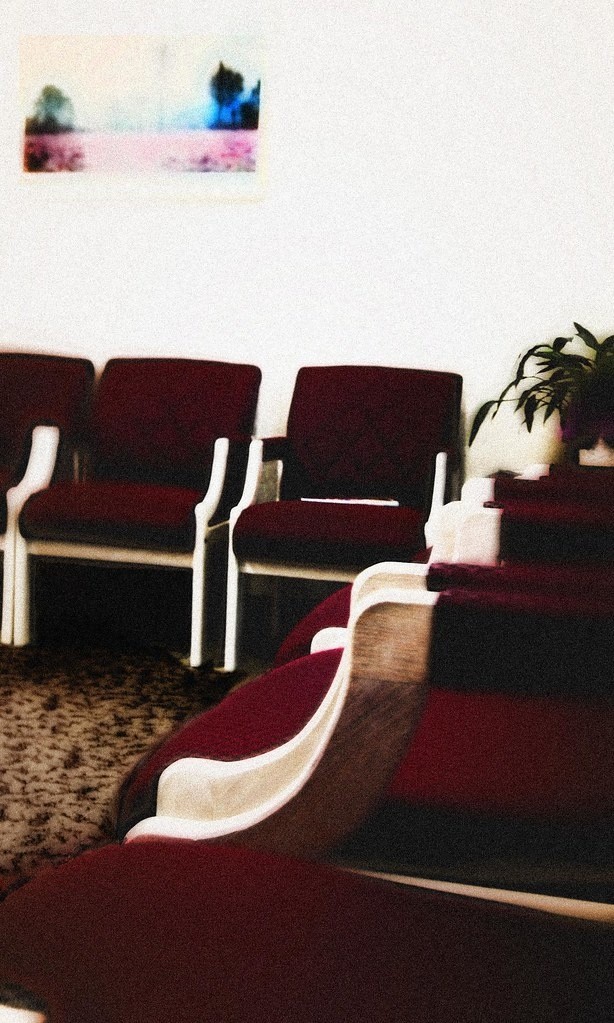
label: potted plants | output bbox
[461,320,614,469]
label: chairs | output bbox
[0,494,614,1023]
[219,367,471,675]
[12,356,261,674]
[0,352,96,648]
